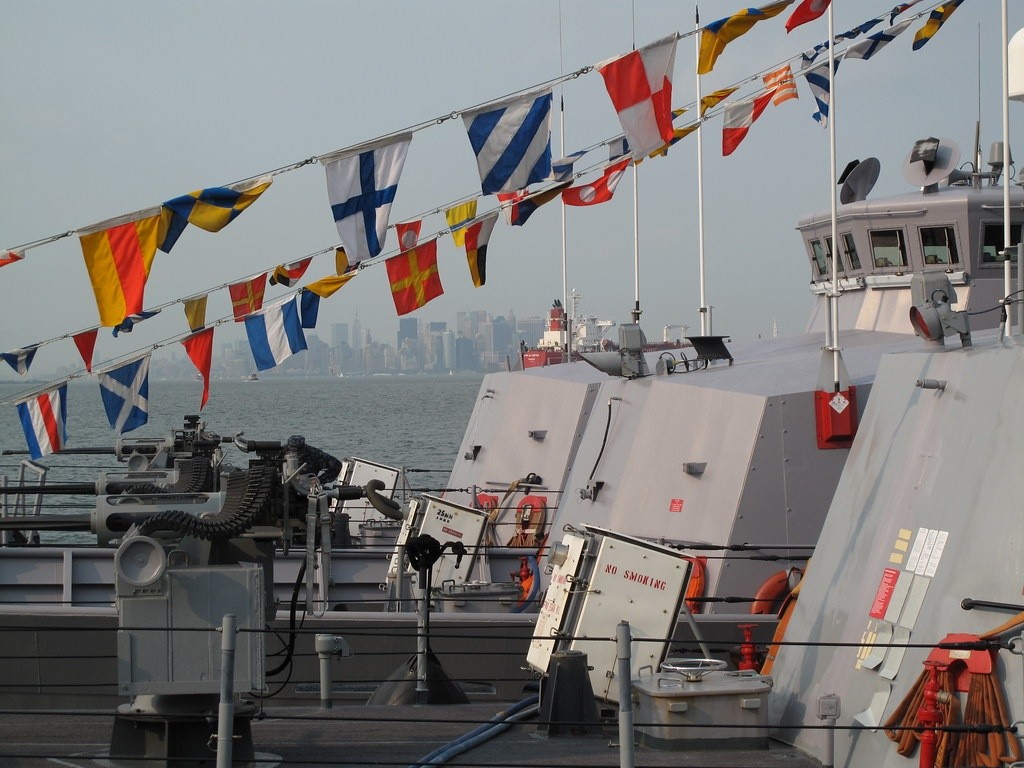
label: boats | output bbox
[2,11,1024,697]
[243,373,260,383]
[194,372,204,381]
[515,289,699,373]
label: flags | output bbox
[2,2,988,459]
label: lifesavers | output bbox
[751,568,806,614]
